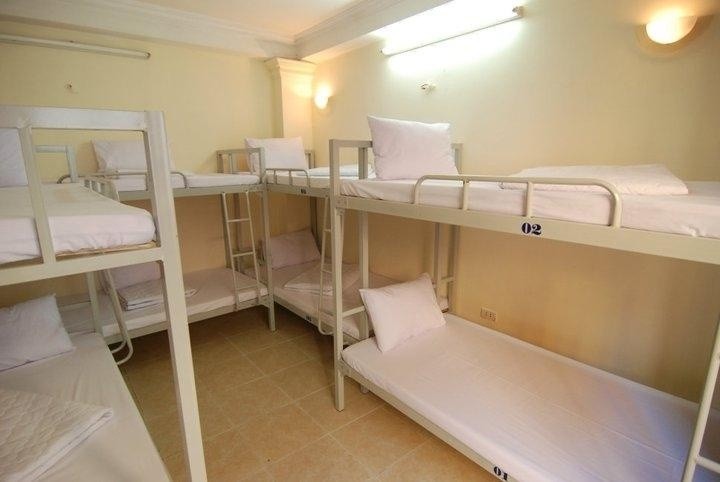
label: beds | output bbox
[1,105,208,482]
[328,138,719,479]
[231,148,462,395]
[44,148,277,346]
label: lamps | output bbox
[645,14,699,46]
[0,32,151,60]
[378,5,524,57]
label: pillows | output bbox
[99,262,159,294]
[359,272,446,354]
[92,139,145,171]
[262,228,321,270]
[1,127,28,188]
[367,115,459,180]
[245,136,307,174]
[0,294,75,370]
